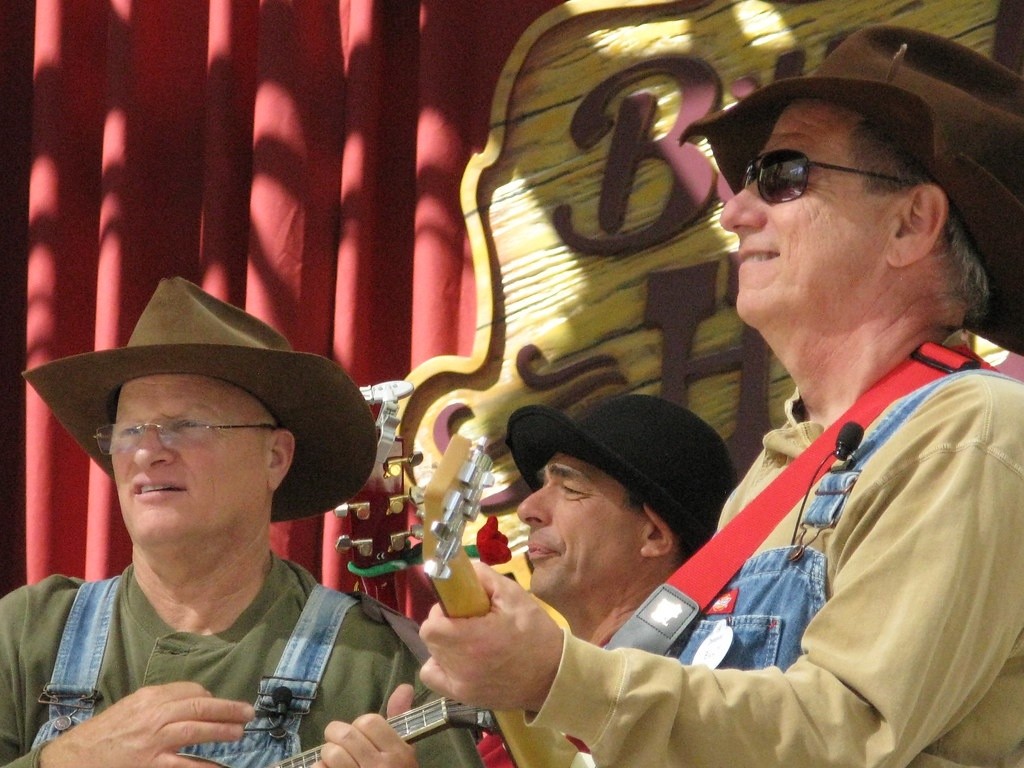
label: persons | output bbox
[418,22,1024,768]
[0,277,488,768]
[477,392,740,768]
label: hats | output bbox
[680,25,1024,358]
[505,392,734,550]
[21,277,378,522]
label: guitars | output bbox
[407,430,598,768]
[334,435,425,614]
[180,694,503,767]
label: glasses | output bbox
[93,414,278,455]
[740,148,955,244]
